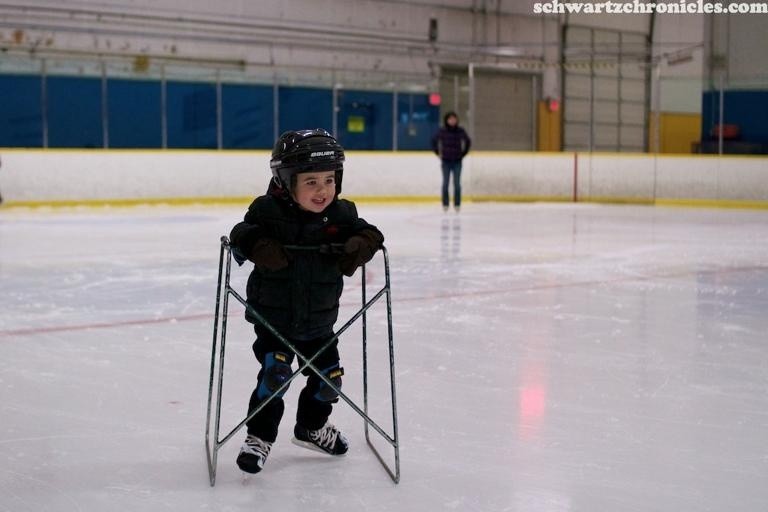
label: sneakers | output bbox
[236,434,272,473]
[443,206,460,210]
[294,421,349,455]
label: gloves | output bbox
[259,242,289,270]
[345,236,371,264]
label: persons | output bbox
[431,110,471,211]
[227,124,387,476]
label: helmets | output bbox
[269,129,345,200]
